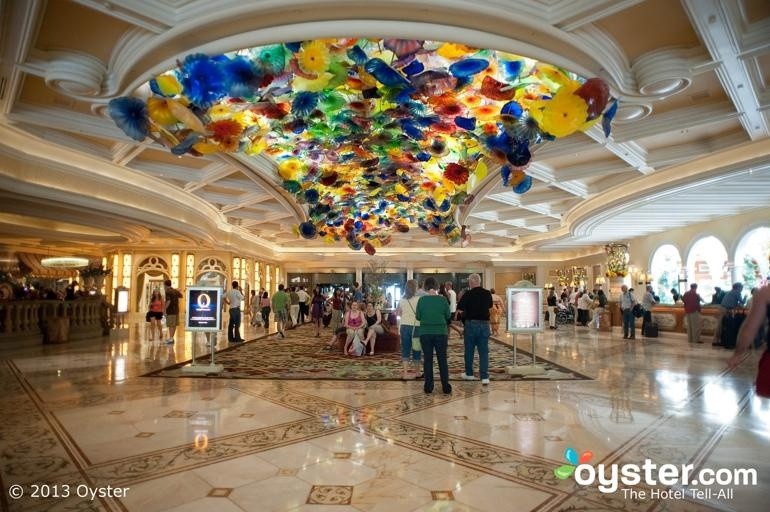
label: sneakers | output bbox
[460,372,475,381]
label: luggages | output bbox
[721,307,748,349]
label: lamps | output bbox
[541,268,657,292]
[605,242,629,279]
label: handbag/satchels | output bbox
[644,322,658,337]
[632,304,642,317]
[348,335,363,357]
[553,308,560,313]
[411,337,422,351]
[374,324,385,336]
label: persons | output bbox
[421,390,494,472]
[222,280,246,343]
[457,272,493,386]
[145,343,176,373]
[416,275,452,394]
[204,330,219,347]
[164,280,183,344]
[726,282,770,400]
[148,288,163,341]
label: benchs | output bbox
[334,310,403,354]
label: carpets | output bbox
[138,318,597,384]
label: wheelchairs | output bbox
[556,300,575,324]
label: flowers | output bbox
[367,258,390,310]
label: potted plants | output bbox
[78,270,96,292]
[90,264,112,292]
